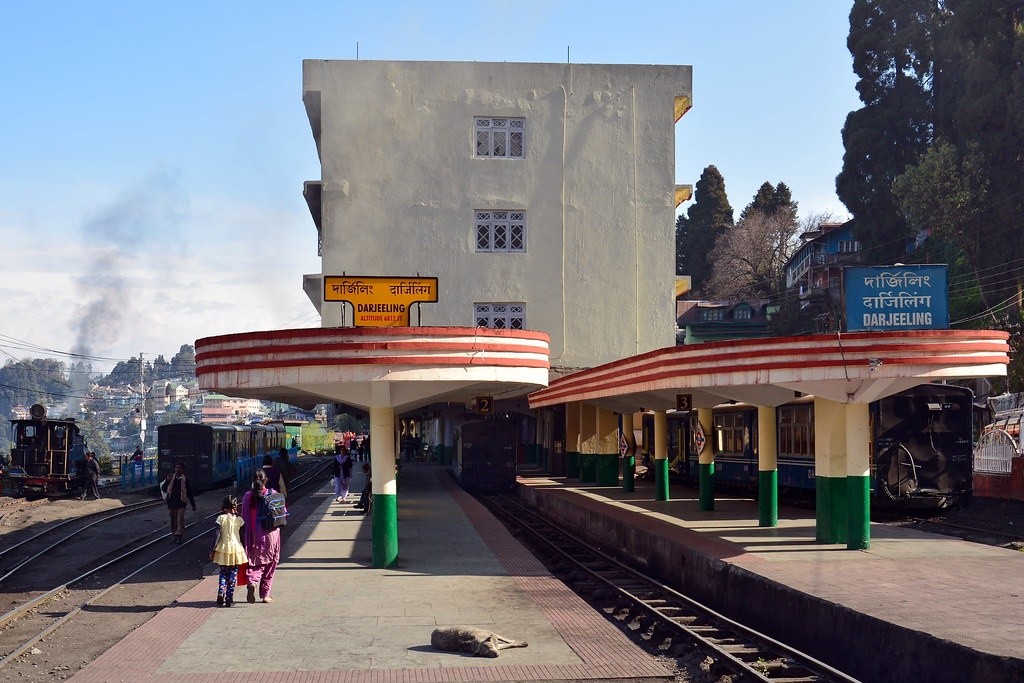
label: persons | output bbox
[162,463,197,545]
[130,446,143,462]
[330,427,430,511]
[76,452,101,500]
[209,426,301,608]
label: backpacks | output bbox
[262,488,286,530]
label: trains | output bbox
[453,419,518,491]
[157,424,286,491]
[643,382,974,512]
[8,403,86,500]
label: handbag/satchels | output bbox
[160,479,167,500]
[330,479,335,486]
[237,562,249,586]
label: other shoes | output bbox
[353,504,364,509]
[226,599,235,607]
[246,584,255,602]
[217,593,224,605]
[172,532,183,544]
[264,595,275,603]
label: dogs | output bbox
[431,626,529,658]
